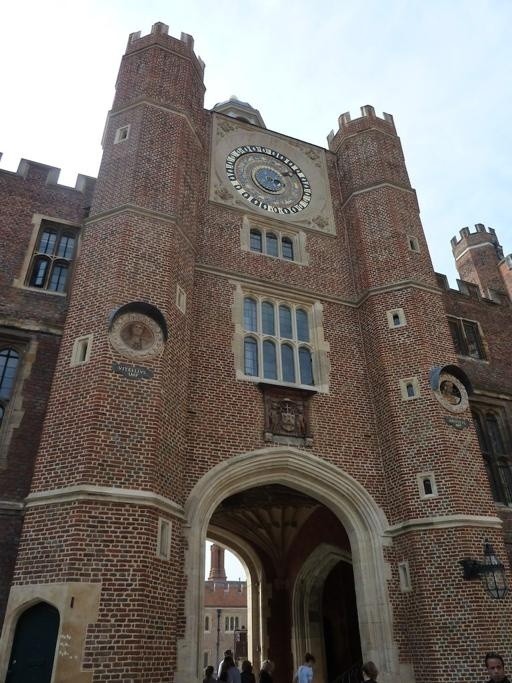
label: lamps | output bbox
[458,538,509,599]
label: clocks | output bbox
[226,145,311,214]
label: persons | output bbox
[361,661,379,682]
[442,381,460,405]
[239,661,256,683]
[123,324,146,351]
[484,652,509,683]
[257,659,276,683]
[218,656,241,683]
[216,650,233,683]
[293,653,316,682]
[269,402,281,431]
[294,409,307,436]
[202,666,218,683]
